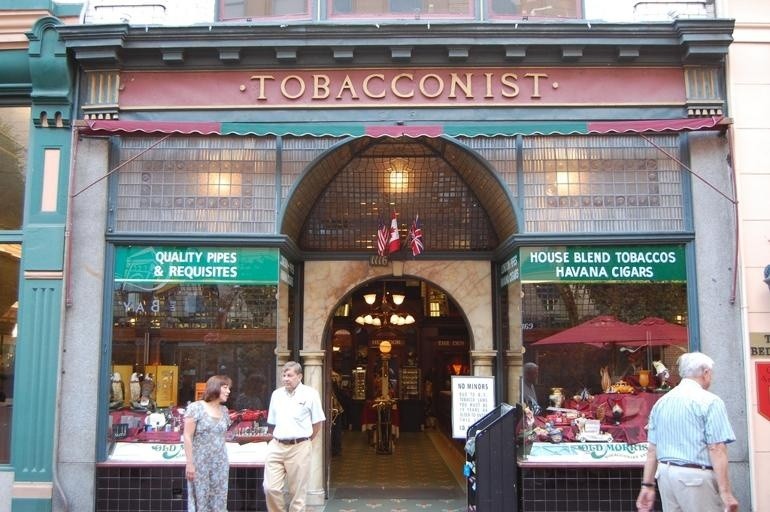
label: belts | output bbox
[660,461,713,470]
[278,438,307,445]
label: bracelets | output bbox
[641,481,657,489]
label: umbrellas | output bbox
[528,314,677,393]
[580,313,689,381]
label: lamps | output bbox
[354,280,416,327]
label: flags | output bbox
[408,211,426,257]
[389,207,400,254]
[377,211,392,259]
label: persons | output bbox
[235,374,265,412]
[634,351,741,512]
[183,374,233,511]
[520,360,539,409]
[262,360,327,511]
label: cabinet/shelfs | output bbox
[464,402,520,512]
